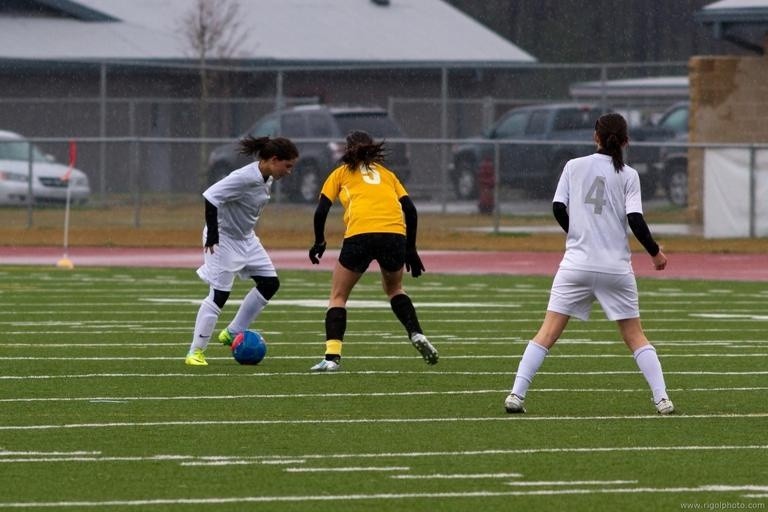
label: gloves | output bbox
[405,249,425,278]
[309,241,326,265]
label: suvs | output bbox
[207,102,411,204]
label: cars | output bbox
[1,131,92,211]
[653,102,689,207]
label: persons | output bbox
[184,134,301,366]
[501,110,675,415]
[307,130,440,371]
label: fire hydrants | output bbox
[477,154,499,215]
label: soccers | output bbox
[231,331,266,365]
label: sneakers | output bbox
[218,328,235,346]
[310,356,340,371]
[184,348,208,366]
[655,399,674,414]
[505,394,527,414]
[410,332,439,366]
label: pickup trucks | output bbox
[446,101,674,200]
